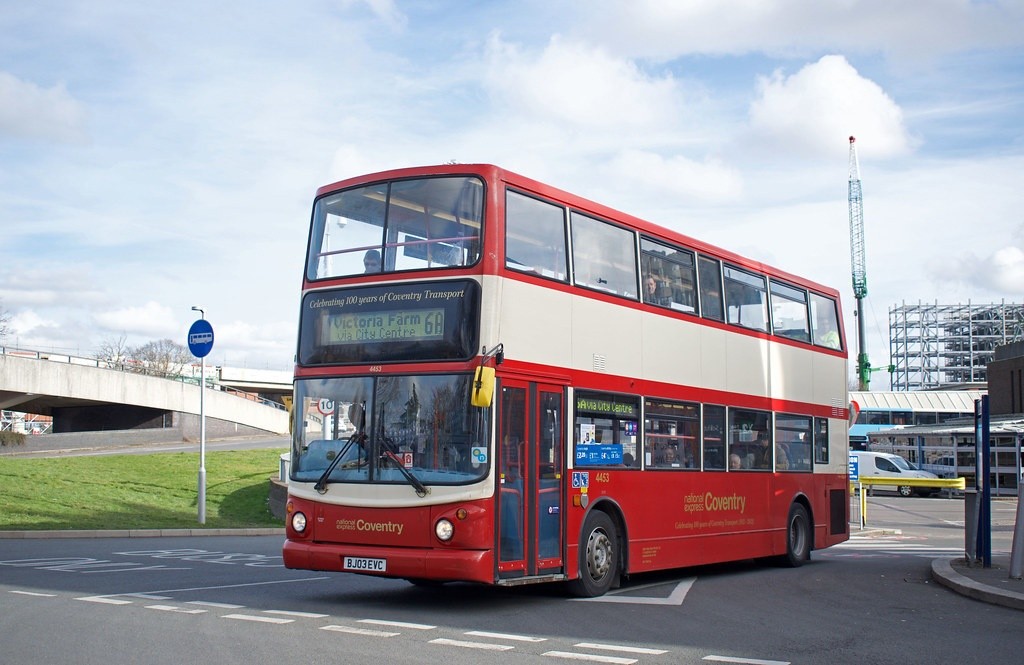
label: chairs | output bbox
[773,329,810,343]
[779,442,795,472]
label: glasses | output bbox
[364,262,378,266]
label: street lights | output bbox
[191,305,206,524]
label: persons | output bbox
[348,404,396,460]
[729,454,745,469]
[364,250,381,273]
[595,430,602,443]
[750,431,789,469]
[700,278,724,320]
[658,447,686,468]
[777,316,841,350]
[643,275,673,308]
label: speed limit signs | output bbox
[317,397,335,414]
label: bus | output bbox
[282,163,856,599]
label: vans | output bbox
[848,450,942,496]
